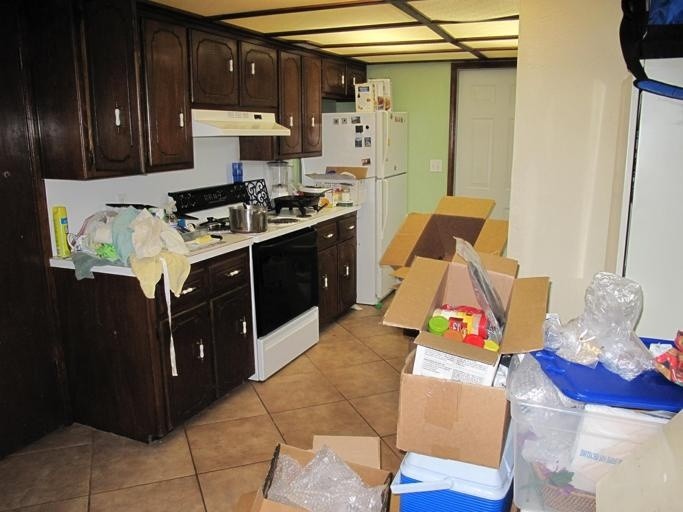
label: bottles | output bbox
[51,206,71,258]
[424,308,500,352]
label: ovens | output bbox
[251,226,318,341]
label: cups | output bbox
[230,161,243,183]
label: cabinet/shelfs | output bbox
[19,2,193,179]
[323,51,369,102]
[207,244,255,398]
[280,41,322,159]
[193,16,279,112]
[52,240,216,441]
[276,207,357,324]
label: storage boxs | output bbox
[398,348,512,463]
[383,195,509,280]
[381,235,550,383]
[507,335,683,512]
[391,419,514,512]
[253,433,391,511]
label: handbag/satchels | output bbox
[619,0,682,100]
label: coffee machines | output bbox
[266,159,293,208]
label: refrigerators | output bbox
[300,110,409,308]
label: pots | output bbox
[227,203,269,233]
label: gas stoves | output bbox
[187,217,306,244]
